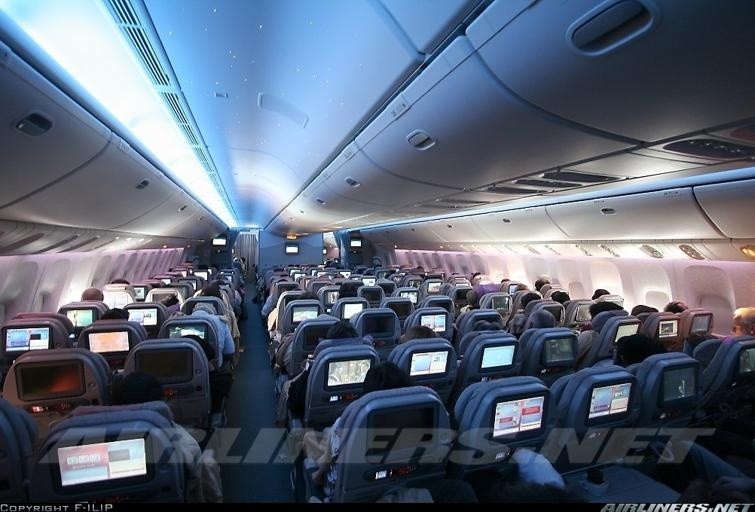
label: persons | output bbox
[0,259,755,503]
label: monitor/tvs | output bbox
[586,379,635,425]
[285,243,299,254]
[661,365,698,402]
[16,360,84,401]
[490,396,544,445]
[5,267,234,354]
[47,433,155,495]
[367,403,439,453]
[136,349,192,383]
[212,235,227,245]
[265,264,755,391]
[350,238,361,246]
